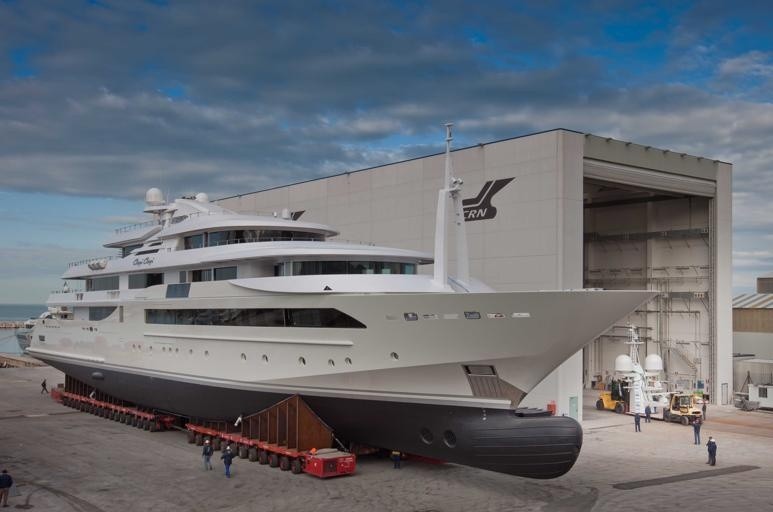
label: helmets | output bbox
[226,446,231,450]
[711,439,715,442]
[204,440,210,444]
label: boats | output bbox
[16,306,72,351]
[11,120,663,481]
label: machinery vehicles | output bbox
[51,373,357,480]
[596,324,703,424]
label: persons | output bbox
[220,446,233,478]
[635,411,641,432]
[0,469,14,507]
[709,439,717,466]
[202,440,213,471]
[693,418,700,444]
[40,378,49,394]
[702,403,706,420]
[705,436,712,464]
[645,406,651,423]
